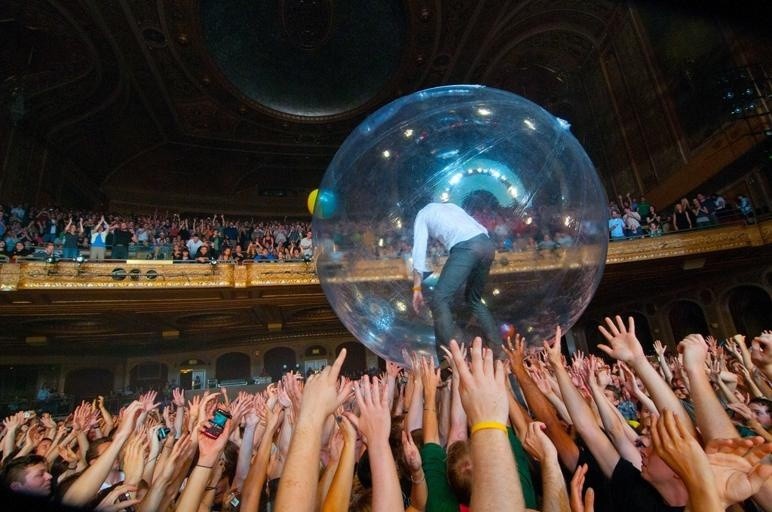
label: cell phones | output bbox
[203,408,231,440]
[119,491,135,512]
[230,497,240,509]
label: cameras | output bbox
[157,427,170,442]
